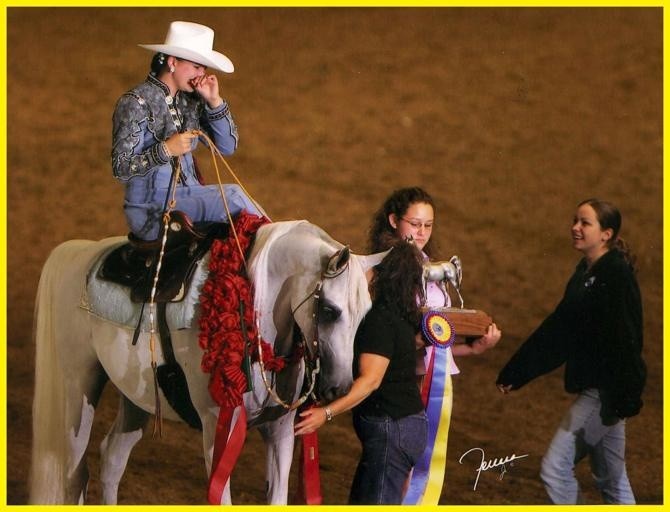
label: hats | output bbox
[138,21,234,74]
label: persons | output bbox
[495,197,648,504]
[293,235,428,505]
[367,186,501,505]
[112,19,268,242]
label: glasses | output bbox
[400,216,434,230]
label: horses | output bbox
[404,234,464,309]
[25,220,395,505]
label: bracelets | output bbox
[162,141,171,158]
[325,405,333,424]
